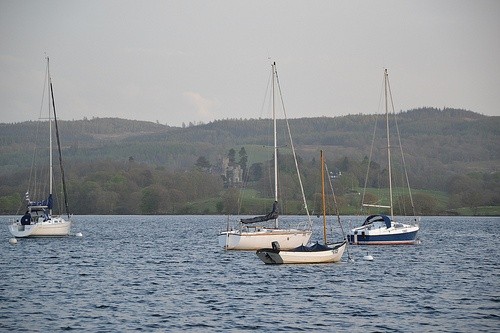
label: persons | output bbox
[25,210,31,219]
[43,208,48,218]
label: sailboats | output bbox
[216,59,313,251]
[7,55,76,237]
[345,68,423,246]
[255,148,347,265]
